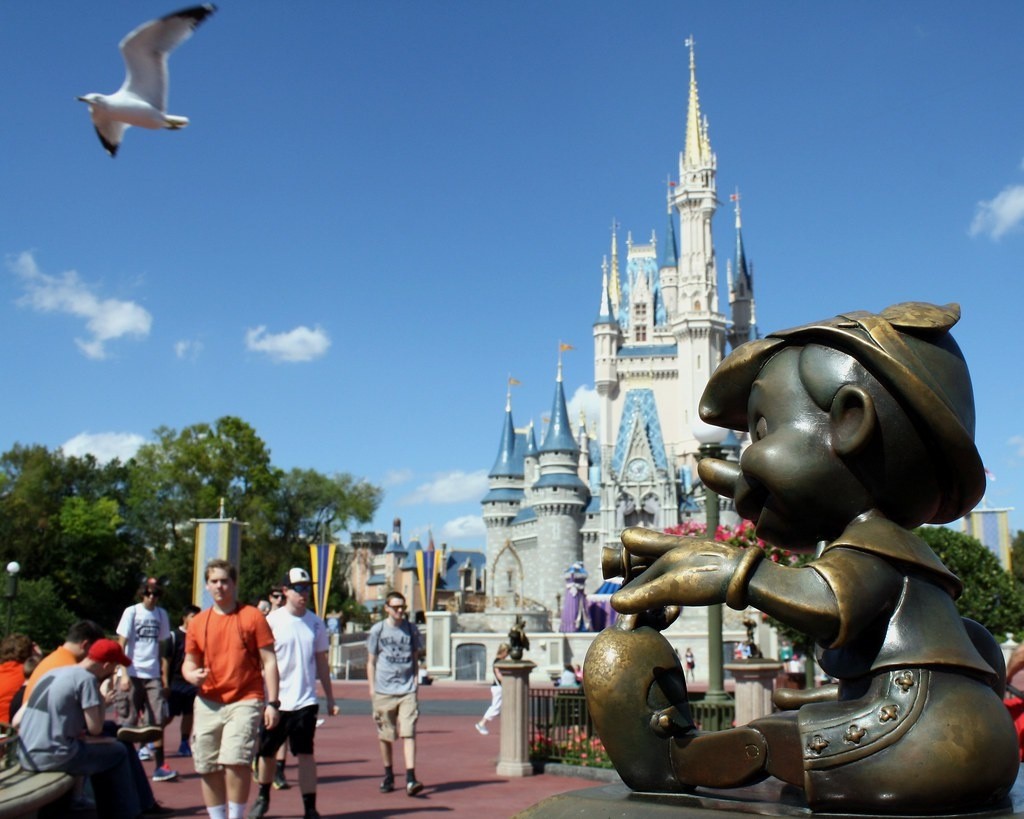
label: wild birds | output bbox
[77,1,218,157]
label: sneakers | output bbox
[147,742,155,749]
[406,780,424,796]
[304,809,320,819]
[380,774,394,792]
[249,795,269,819]
[152,765,178,782]
[178,743,191,755]
[252,761,258,783]
[272,772,288,790]
[137,747,149,761]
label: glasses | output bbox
[140,589,160,597]
[287,584,311,593]
[389,605,407,612]
[270,593,285,599]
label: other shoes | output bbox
[474,723,489,734]
[69,793,97,811]
[140,800,177,817]
[116,723,163,742]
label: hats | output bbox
[145,578,162,590]
[283,567,310,586]
[88,638,132,667]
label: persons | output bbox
[474,643,511,735]
[1002,635,1024,819]
[0,560,425,819]
[734,641,744,666]
[685,648,695,682]
[674,648,681,660]
[560,664,583,689]
[779,639,809,672]
